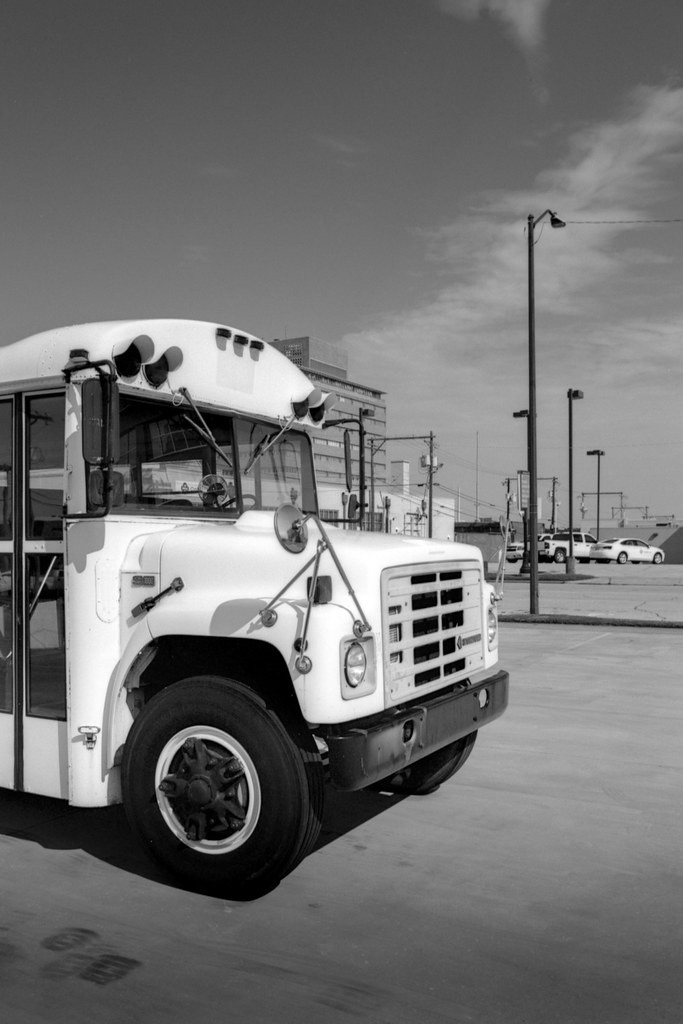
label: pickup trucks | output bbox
[527,531,599,563]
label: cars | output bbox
[589,538,666,564]
[505,533,554,563]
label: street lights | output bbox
[359,410,375,530]
[587,449,605,543]
[342,491,348,529]
[421,500,426,538]
[567,388,584,557]
[385,497,390,534]
[510,210,566,615]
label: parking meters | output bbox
[291,488,298,508]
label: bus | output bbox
[0,319,510,893]
